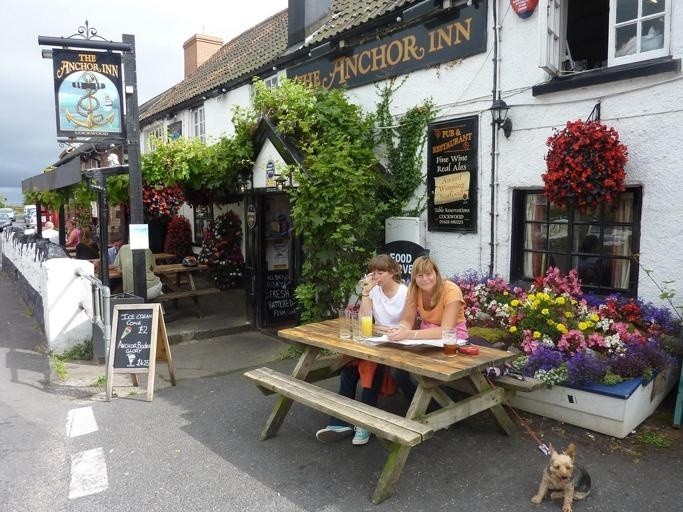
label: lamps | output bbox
[488,90,512,139]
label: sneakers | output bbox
[352,426,370,445]
[316,425,353,444]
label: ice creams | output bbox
[120,326,131,340]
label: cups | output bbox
[339,309,351,338]
[360,310,373,337]
[442,327,457,357]
[351,311,361,342]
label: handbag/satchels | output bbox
[485,365,509,378]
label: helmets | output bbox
[183,256,198,267]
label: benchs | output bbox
[243,366,433,448]
[64,246,221,324]
[489,373,547,393]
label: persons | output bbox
[113,239,165,316]
[315,254,409,447]
[42,222,59,245]
[107,232,126,269]
[64,221,80,248]
[385,256,468,414]
[76,229,97,259]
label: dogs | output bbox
[530,441,593,512]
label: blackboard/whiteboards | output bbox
[265,269,301,324]
[110,303,159,374]
